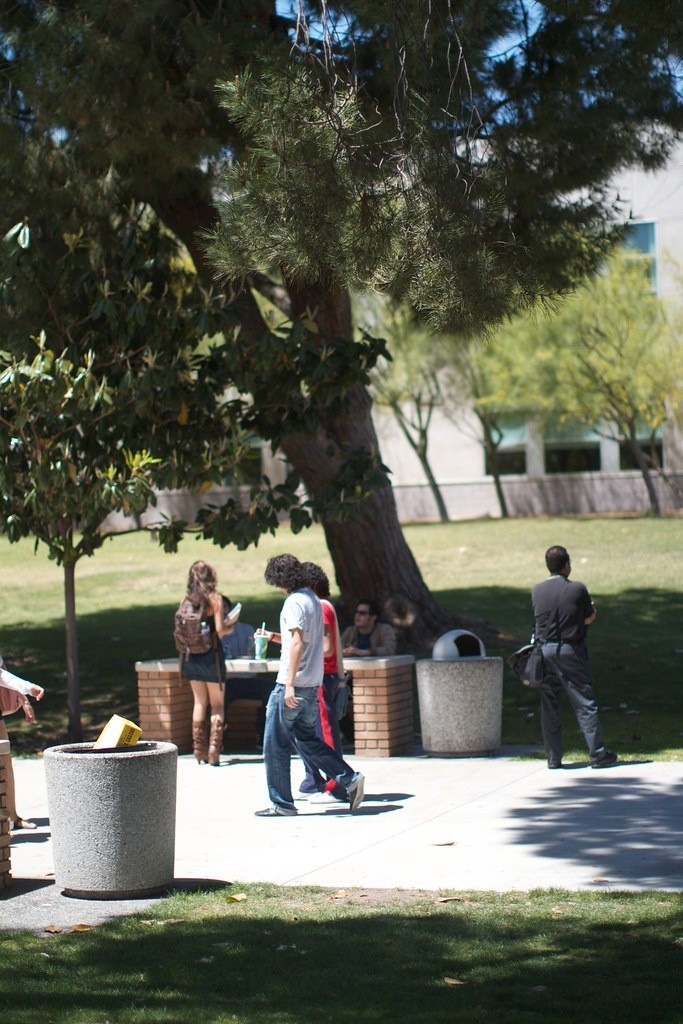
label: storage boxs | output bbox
[92,714,143,749]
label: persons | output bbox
[0,655,45,828]
[182,560,232,767]
[215,554,396,816]
[532,546,618,768]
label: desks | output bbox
[134,654,416,758]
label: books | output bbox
[224,602,242,626]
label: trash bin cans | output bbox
[41,740,178,899]
[413,656,506,762]
[427,628,489,659]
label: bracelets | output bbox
[270,633,275,642]
[23,701,30,704]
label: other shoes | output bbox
[308,790,348,803]
[290,787,319,800]
[344,771,365,811]
[255,806,298,816]
[547,761,562,768]
[591,751,618,768]
[10,817,37,829]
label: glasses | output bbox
[355,610,371,615]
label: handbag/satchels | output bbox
[507,644,544,688]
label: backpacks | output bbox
[174,596,213,654]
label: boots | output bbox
[192,720,208,765]
[208,718,228,766]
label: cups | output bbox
[254,635,269,659]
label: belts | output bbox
[547,640,579,644]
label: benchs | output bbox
[222,699,355,755]
